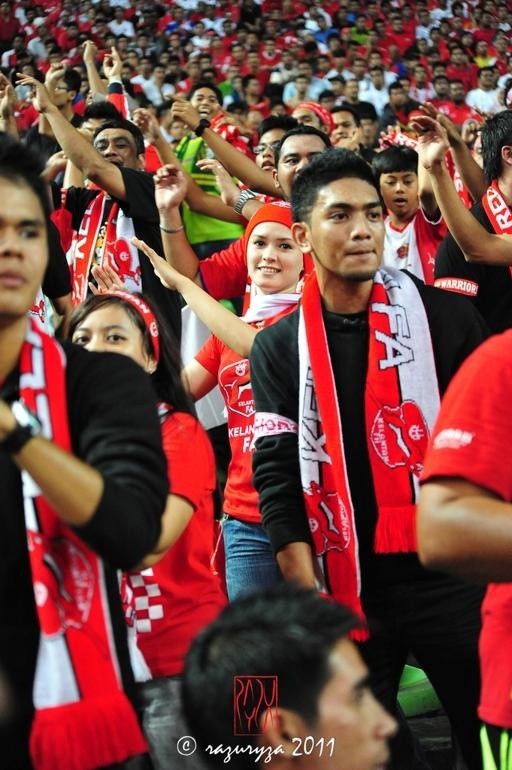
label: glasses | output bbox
[252,143,281,155]
[54,85,70,91]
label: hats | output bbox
[327,74,346,85]
